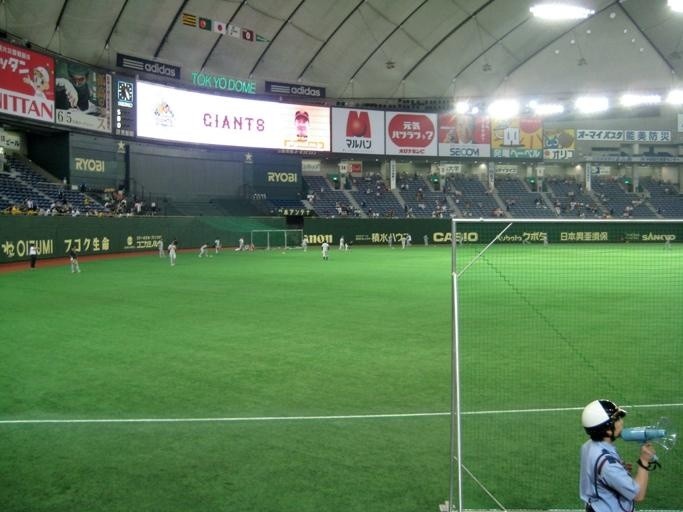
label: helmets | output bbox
[581,399,627,431]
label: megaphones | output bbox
[620,416,678,462]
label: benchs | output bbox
[0,147,683,218]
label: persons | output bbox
[198,243,210,258]
[422,234,429,248]
[214,236,224,253]
[168,240,178,266]
[338,236,344,250]
[0,146,6,161]
[387,232,393,248]
[29,243,40,269]
[397,235,407,249]
[0,175,162,217]
[577,399,657,512]
[56,60,103,118]
[69,246,80,273]
[157,238,166,258]
[305,170,683,221]
[233,234,244,252]
[405,232,411,247]
[301,234,309,250]
[320,239,330,261]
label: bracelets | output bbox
[637,458,651,469]
